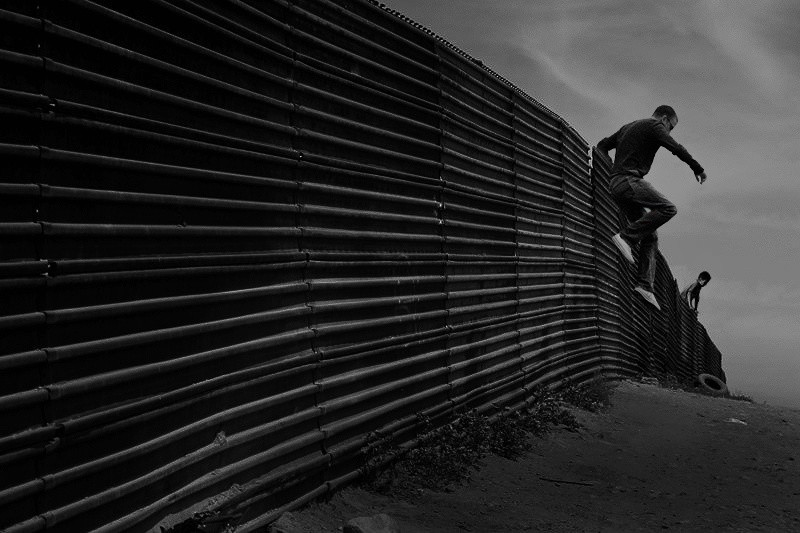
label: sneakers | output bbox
[634,285,662,311]
[612,232,636,264]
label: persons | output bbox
[681,271,712,314]
[597,105,707,311]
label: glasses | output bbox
[667,116,677,131]
